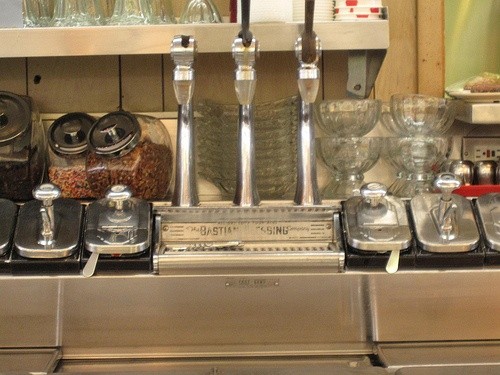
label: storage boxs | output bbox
[86,111,174,203]
[45,112,96,200]
[0,90,47,201]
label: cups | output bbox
[291,0,385,22]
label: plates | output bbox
[446,92,500,103]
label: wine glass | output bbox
[314,94,457,199]
[20,0,224,29]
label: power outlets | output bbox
[463,138,500,164]
